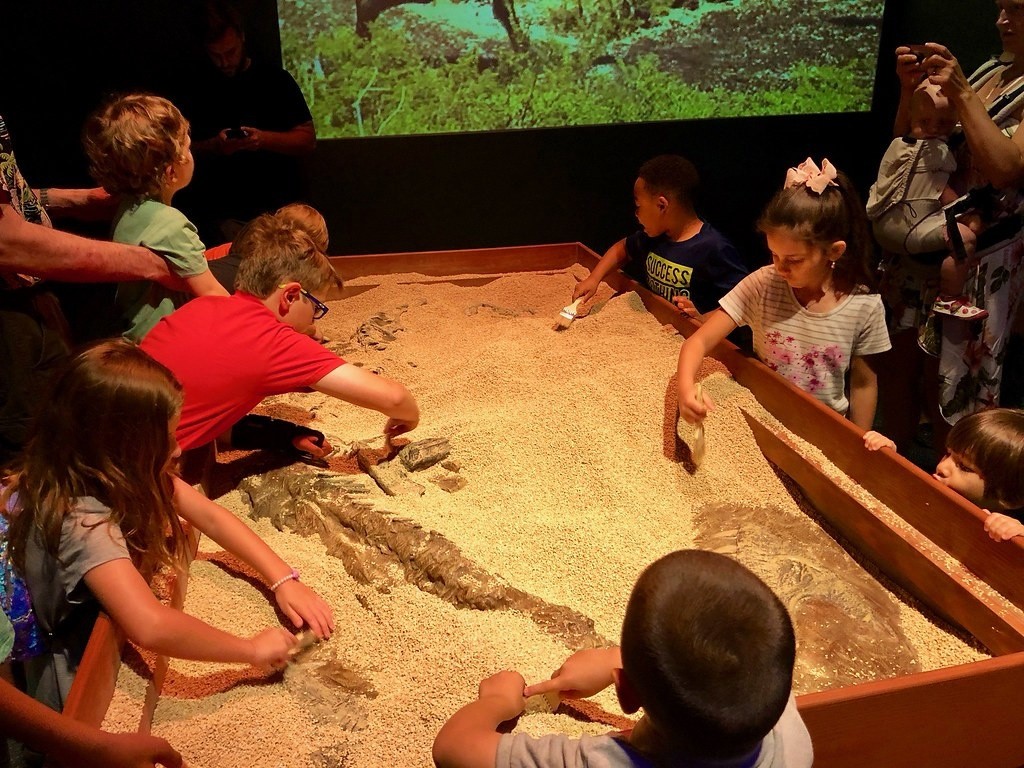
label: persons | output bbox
[864,409,1024,543]
[433,549,815,768]
[895,1,1024,474]
[137,201,418,453]
[0,336,336,698]
[186,5,316,236]
[677,156,893,433]
[865,74,990,357]
[573,152,755,358]
[88,95,232,345]
[0,112,188,294]
[0,606,188,768]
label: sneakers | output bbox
[900,308,921,329]
[932,291,989,322]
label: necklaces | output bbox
[796,286,831,309]
[985,65,1024,109]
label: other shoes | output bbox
[914,423,934,447]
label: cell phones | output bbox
[908,45,938,62]
[225,130,249,139]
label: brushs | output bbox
[288,630,316,649]
[554,295,585,329]
[689,381,710,471]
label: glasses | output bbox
[278,284,329,319]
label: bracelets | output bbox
[271,571,300,591]
